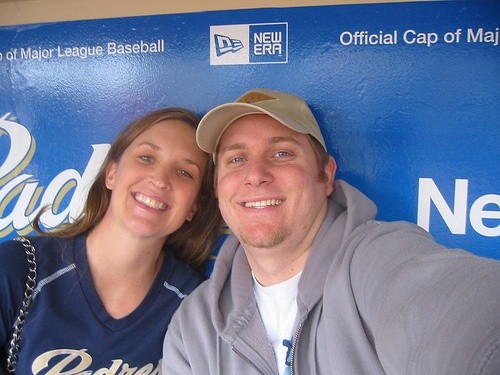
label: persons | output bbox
[0,107,221,375]
[161,87,500,375]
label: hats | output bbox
[195,89,326,165]
